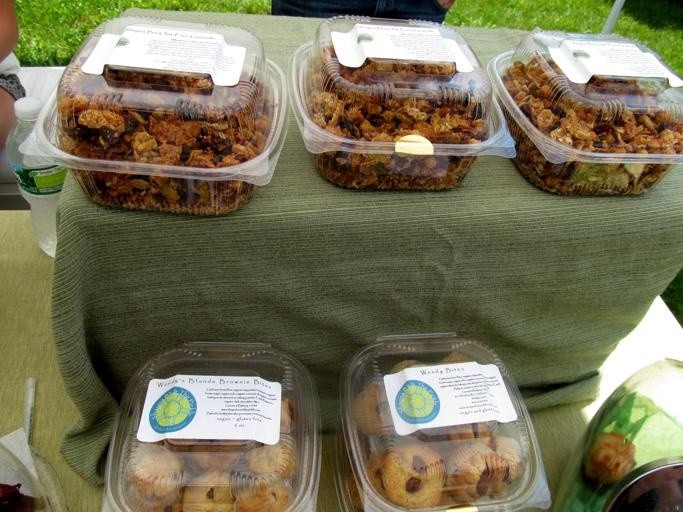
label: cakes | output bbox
[353,351,526,509]
[130,398,296,512]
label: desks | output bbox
[1,207,683,512]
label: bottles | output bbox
[5,95,69,259]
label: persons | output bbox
[1,0,24,150]
[270,0,458,24]
